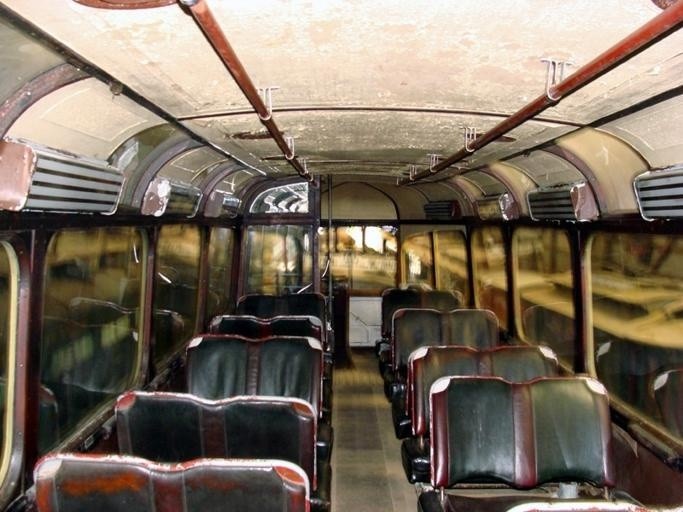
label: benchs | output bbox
[375,285,645,510]
[33,291,333,512]
